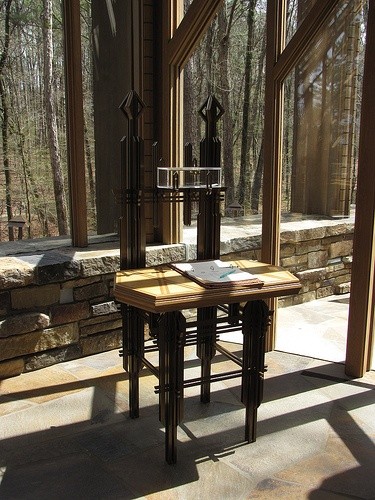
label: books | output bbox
[168,259,265,288]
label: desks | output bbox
[113,258,302,465]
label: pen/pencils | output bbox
[220,269,236,279]
